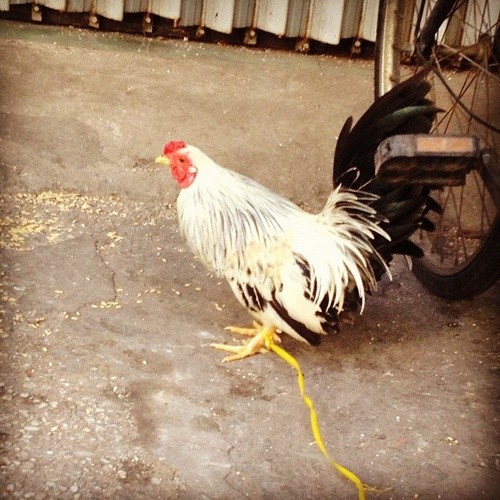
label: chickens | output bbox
[155,62,446,363]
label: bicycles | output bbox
[370,0,500,299]
